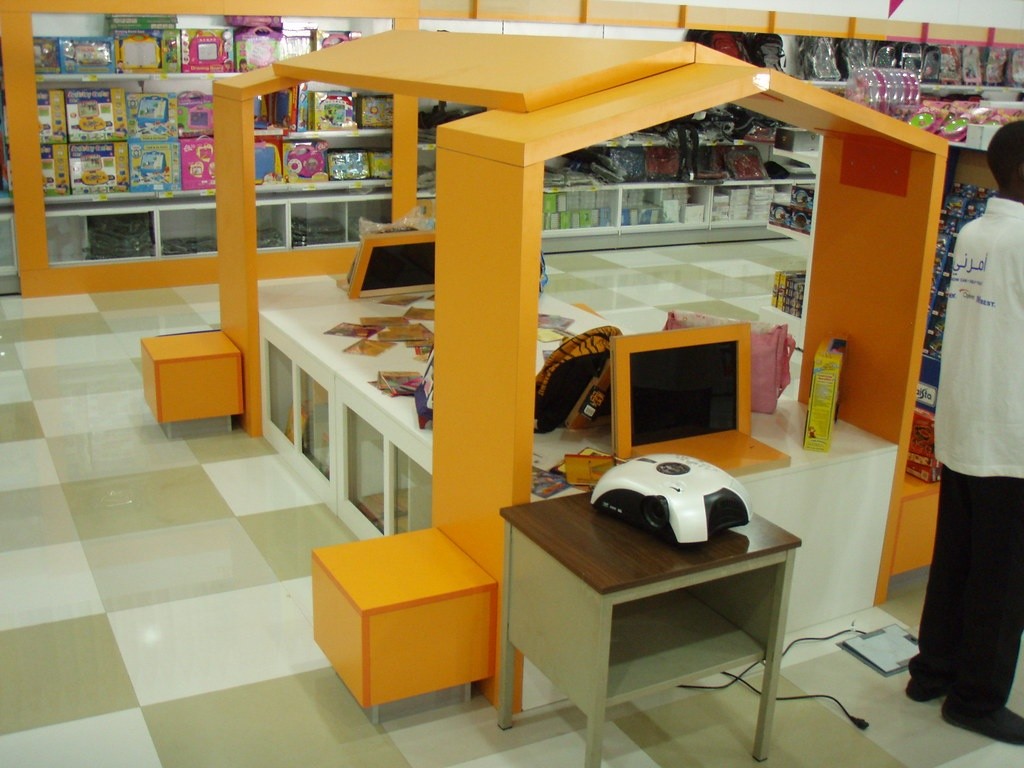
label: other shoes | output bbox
[906,675,947,702]
[942,697,1024,746]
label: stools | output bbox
[313,527,499,725]
[890,470,941,576]
[141,331,245,440]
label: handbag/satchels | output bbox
[663,310,797,414]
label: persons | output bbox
[905,121,1024,748]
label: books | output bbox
[543,187,789,230]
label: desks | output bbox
[498,487,801,768]
[260,293,898,715]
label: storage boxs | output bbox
[713,187,791,220]
[621,187,704,226]
[415,343,433,430]
[906,407,943,483]
[235,27,362,72]
[31,14,235,73]
[544,191,611,230]
[804,336,847,453]
[772,270,806,318]
[967,124,1003,151]
[769,185,814,235]
[0,81,395,196]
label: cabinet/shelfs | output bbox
[767,100,1024,414]
[0,69,1024,295]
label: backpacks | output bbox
[686,29,1024,88]
[536,325,624,433]
